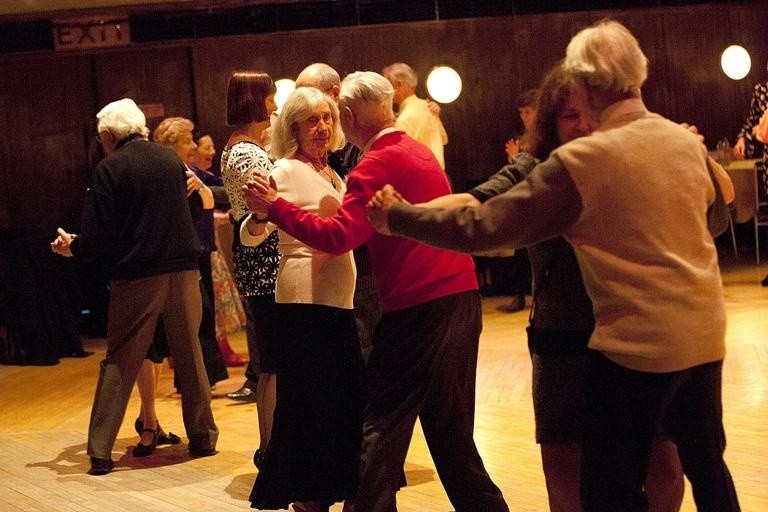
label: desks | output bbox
[703,150,767,261]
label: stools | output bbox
[724,201,741,266]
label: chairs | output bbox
[746,159,767,269]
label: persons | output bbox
[49,19,768,512]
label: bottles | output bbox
[717,137,732,162]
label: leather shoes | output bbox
[91,457,114,472]
[133,418,179,457]
[224,387,256,401]
